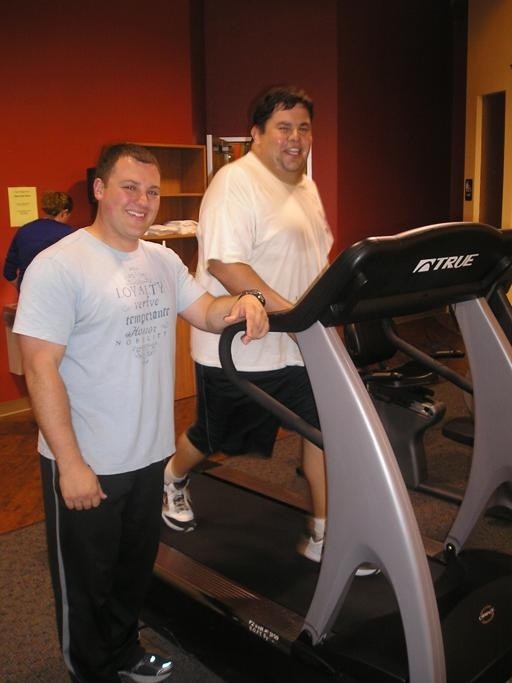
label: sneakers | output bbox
[116,651,176,682]
[303,537,381,576]
[161,475,198,533]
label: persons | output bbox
[161,89,381,578]
[4,192,74,293]
[10,145,271,683]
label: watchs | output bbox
[237,290,266,306]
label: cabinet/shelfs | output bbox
[99,140,237,403]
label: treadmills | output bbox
[152,222,511,681]
